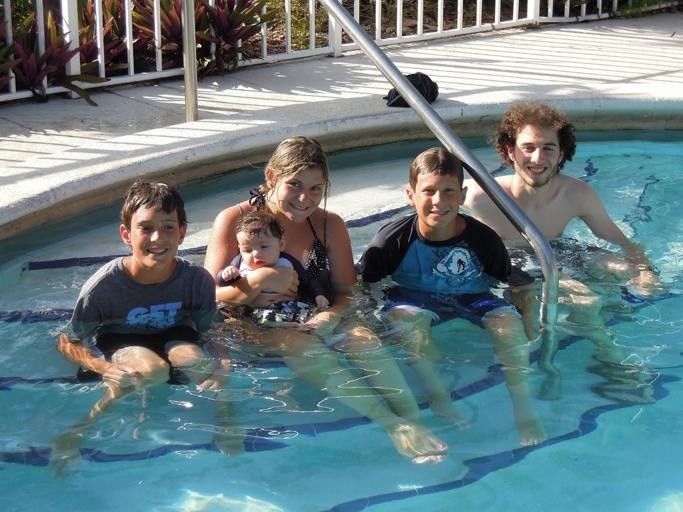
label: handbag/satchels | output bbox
[383,72,439,107]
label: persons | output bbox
[215,212,346,346]
[354,146,547,447]
[204,136,449,466]
[459,101,662,403]
[50,179,243,477]
